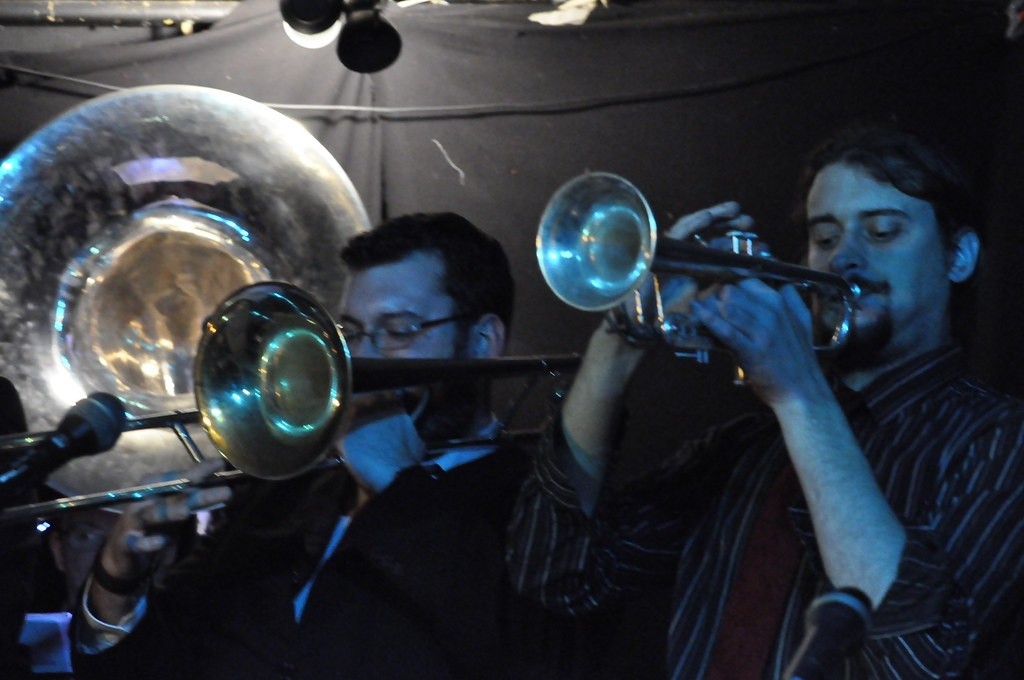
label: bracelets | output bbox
[93,541,136,595]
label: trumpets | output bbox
[534,169,862,370]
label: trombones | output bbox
[0,278,583,520]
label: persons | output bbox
[71,212,624,680]
[0,377,117,615]
[504,139,1024,680]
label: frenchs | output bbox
[0,82,374,518]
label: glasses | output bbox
[336,315,478,360]
